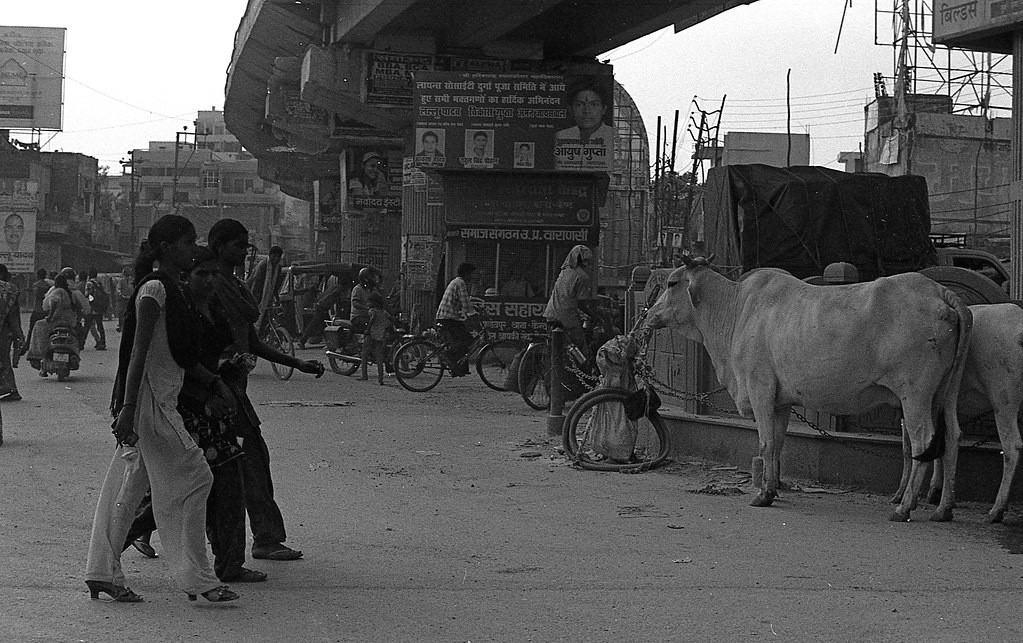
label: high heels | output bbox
[184,585,240,601]
[85,578,144,603]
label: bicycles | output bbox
[257,305,295,379]
[394,304,524,393]
[517,311,619,411]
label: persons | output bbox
[357,290,391,385]
[469,130,487,163]
[121,243,266,582]
[246,245,283,306]
[131,219,326,560]
[515,143,532,167]
[542,244,607,386]
[555,78,613,169]
[416,131,443,166]
[348,150,386,207]
[85,213,239,602]
[3,213,23,252]
[435,263,478,375]
[350,267,370,328]
[0,263,135,402]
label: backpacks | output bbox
[89,280,108,312]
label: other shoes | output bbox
[216,567,268,582]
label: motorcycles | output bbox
[325,304,426,378]
[277,263,381,345]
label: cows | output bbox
[891,301,1023,523]
[643,254,974,523]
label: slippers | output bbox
[252,545,303,560]
[131,539,159,558]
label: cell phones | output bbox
[111,418,140,447]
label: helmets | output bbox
[63,267,76,281]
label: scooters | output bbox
[44,294,95,381]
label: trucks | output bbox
[705,163,1010,298]
